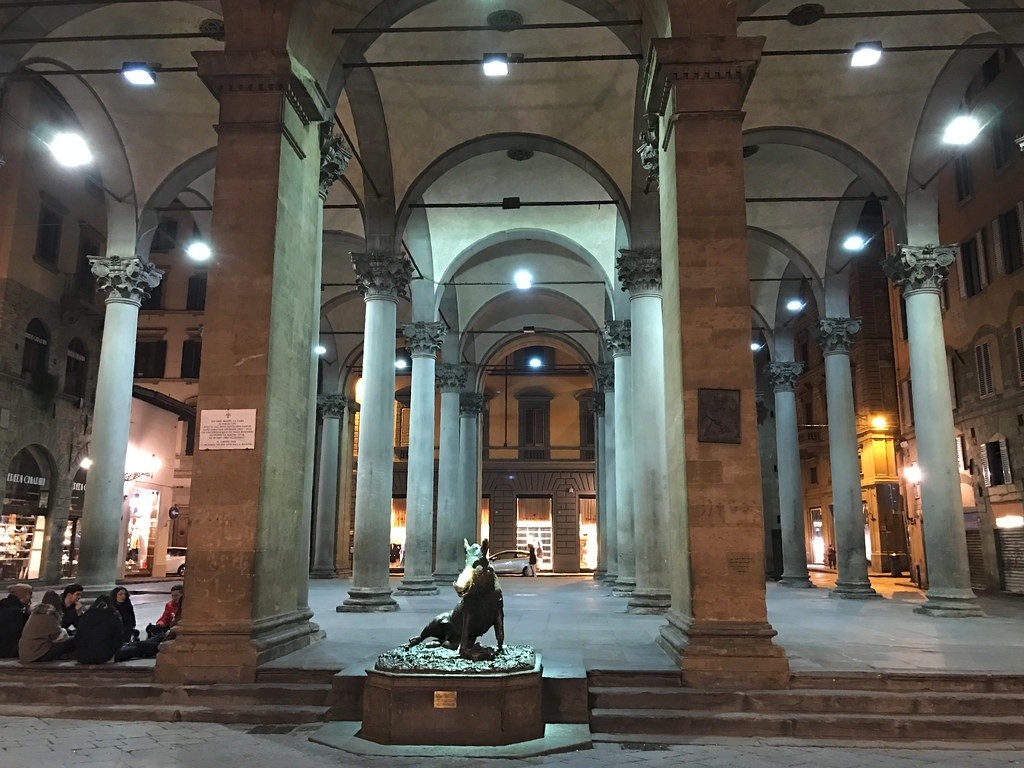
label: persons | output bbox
[526,544,537,578]
[828,544,837,570]
[536,542,543,571]
[0,583,184,665]
[388,543,406,568]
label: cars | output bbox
[166,546,187,577]
[487,550,539,577]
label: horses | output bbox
[405,537,505,658]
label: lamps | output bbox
[850,40,884,67]
[482,52,509,76]
[121,61,156,86]
